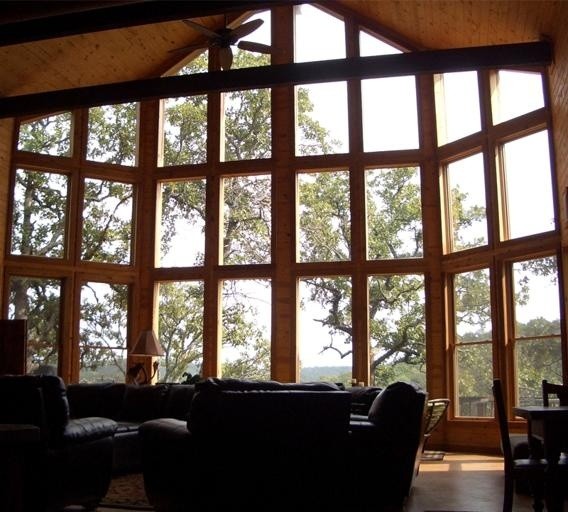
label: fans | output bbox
[166,1,290,60]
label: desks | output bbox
[510,404,568,510]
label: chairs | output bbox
[491,377,568,512]
[540,377,568,408]
[417,396,452,462]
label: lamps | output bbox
[217,44,235,71]
[128,328,166,385]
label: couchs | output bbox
[0,373,426,512]
